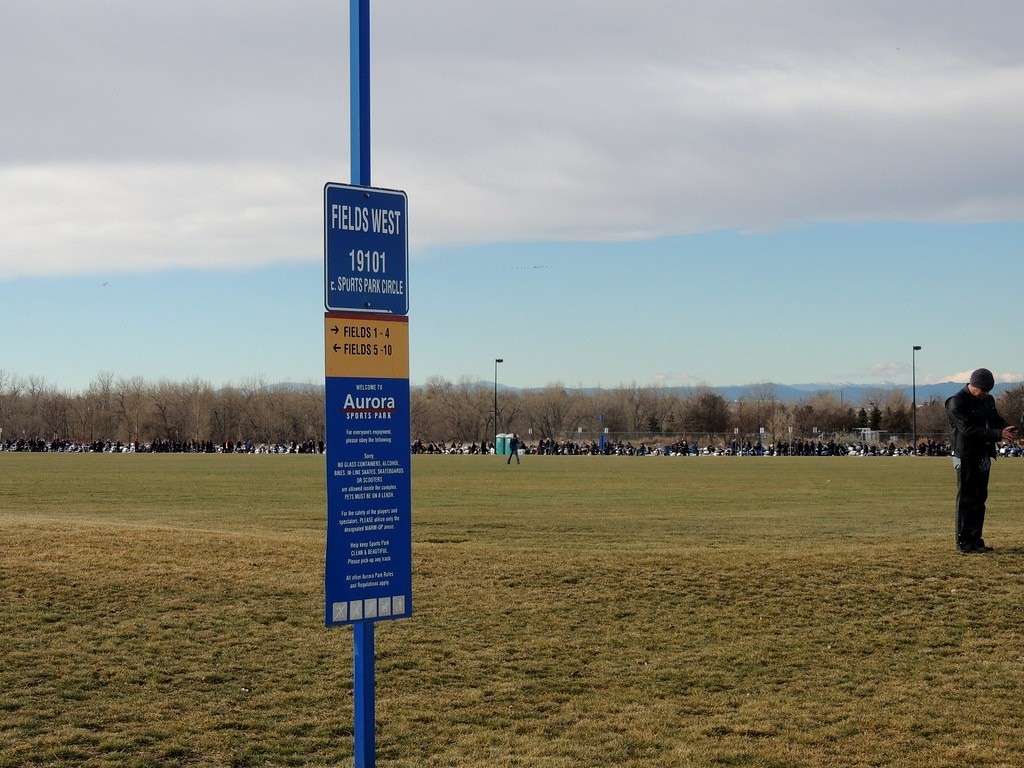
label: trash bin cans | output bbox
[505,433,520,455]
[496,433,508,455]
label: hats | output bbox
[970,368,994,391]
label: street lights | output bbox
[913,345,921,456]
[494,358,503,455]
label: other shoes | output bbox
[962,547,986,554]
[982,546,994,551]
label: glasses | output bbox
[981,389,990,393]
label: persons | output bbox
[218,438,495,455]
[519,438,690,456]
[690,439,1024,457]
[0,437,216,453]
[945,368,1018,553]
[506,434,520,464]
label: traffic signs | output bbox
[324,312,411,626]
